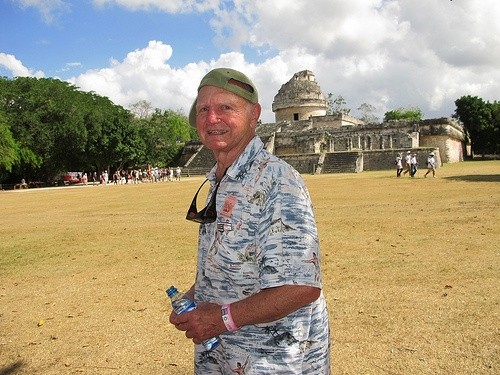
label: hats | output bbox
[430,152,434,155]
[105,171,107,172]
[411,153,416,156]
[189,68,258,128]
[407,151,411,154]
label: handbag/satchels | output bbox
[394,160,398,165]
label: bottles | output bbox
[166,286,223,353]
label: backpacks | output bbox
[425,160,428,166]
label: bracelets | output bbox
[221,304,243,332]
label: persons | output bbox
[395,152,403,177]
[403,151,411,176]
[411,152,417,177]
[61,165,181,186]
[424,152,436,178]
[169,68,331,375]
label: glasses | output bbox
[186,173,226,224]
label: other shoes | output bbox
[403,172,405,176]
[424,175,426,178]
[433,176,436,178]
[409,174,411,176]
[397,175,400,177]
[410,175,415,177]
